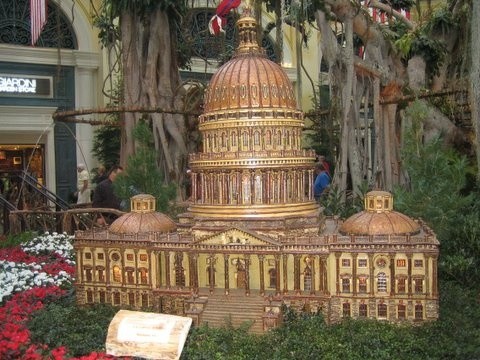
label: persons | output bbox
[318,156,331,175]
[312,162,330,204]
[90,166,109,203]
[94,163,126,229]
[76,164,93,221]
[183,169,194,198]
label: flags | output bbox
[31,0,45,47]
[357,0,412,62]
